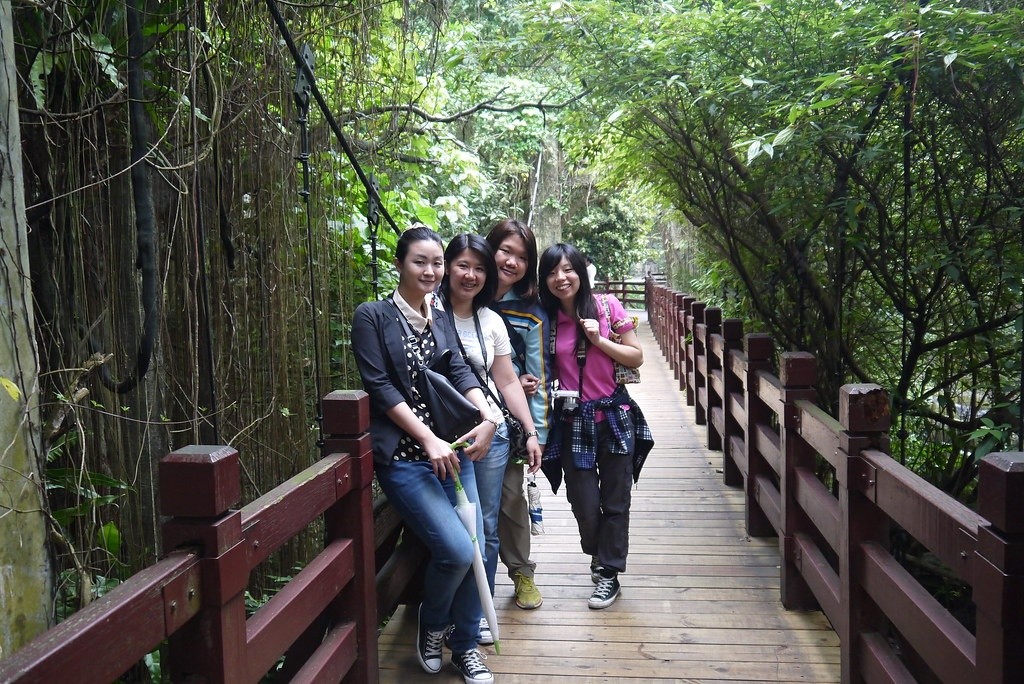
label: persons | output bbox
[349,218,655,684]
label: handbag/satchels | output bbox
[418,366,483,442]
[498,406,524,459]
[600,294,640,383]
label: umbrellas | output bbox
[447,441,500,656]
[526,475,545,536]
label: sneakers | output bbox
[450,648,494,684]
[476,618,494,644]
[511,572,543,609]
[416,602,448,674]
[588,572,621,608]
[591,553,603,584]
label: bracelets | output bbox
[526,430,539,438]
[483,419,499,431]
